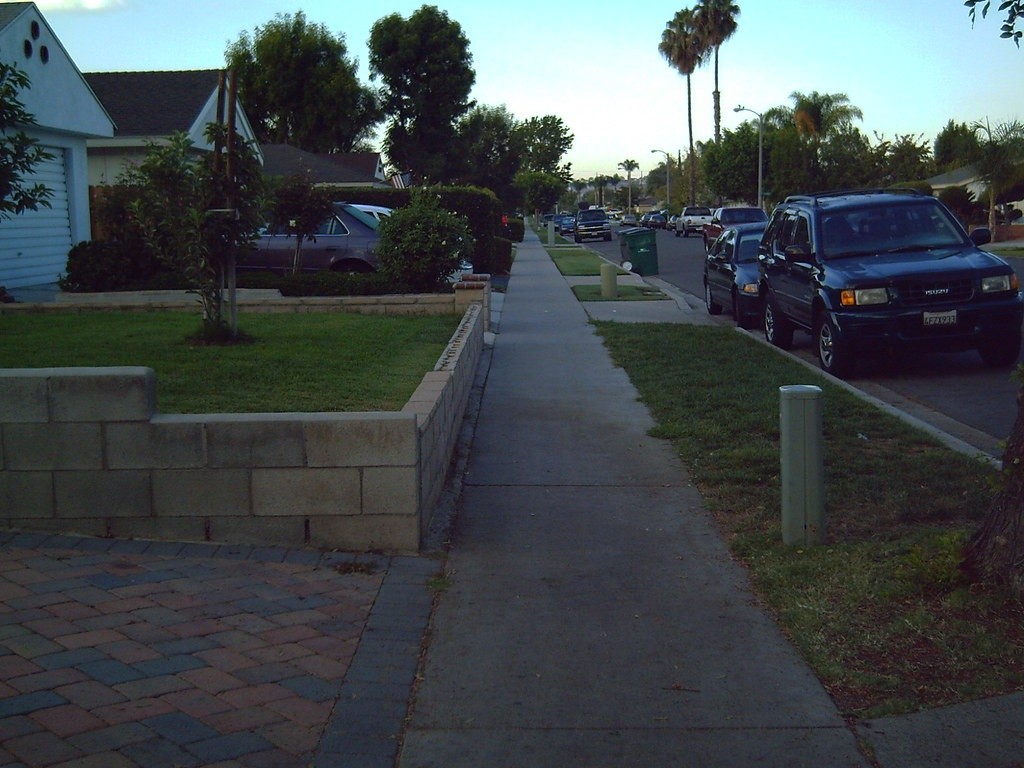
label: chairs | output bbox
[740,239,760,263]
[821,217,858,251]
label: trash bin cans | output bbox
[614,226,659,276]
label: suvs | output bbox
[704,207,769,256]
[570,208,613,243]
[543,213,568,232]
[756,186,1021,376]
[676,206,714,238]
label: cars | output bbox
[597,207,623,220]
[346,201,474,285]
[703,222,773,330]
[559,217,578,236]
[220,200,419,275]
[647,214,666,229]
[638,215,651,228]
[501,212,524,227]
[665,214,679,231]
[619,214,638,227]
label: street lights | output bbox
[736,104,763,209]
[651,149,670,224]
